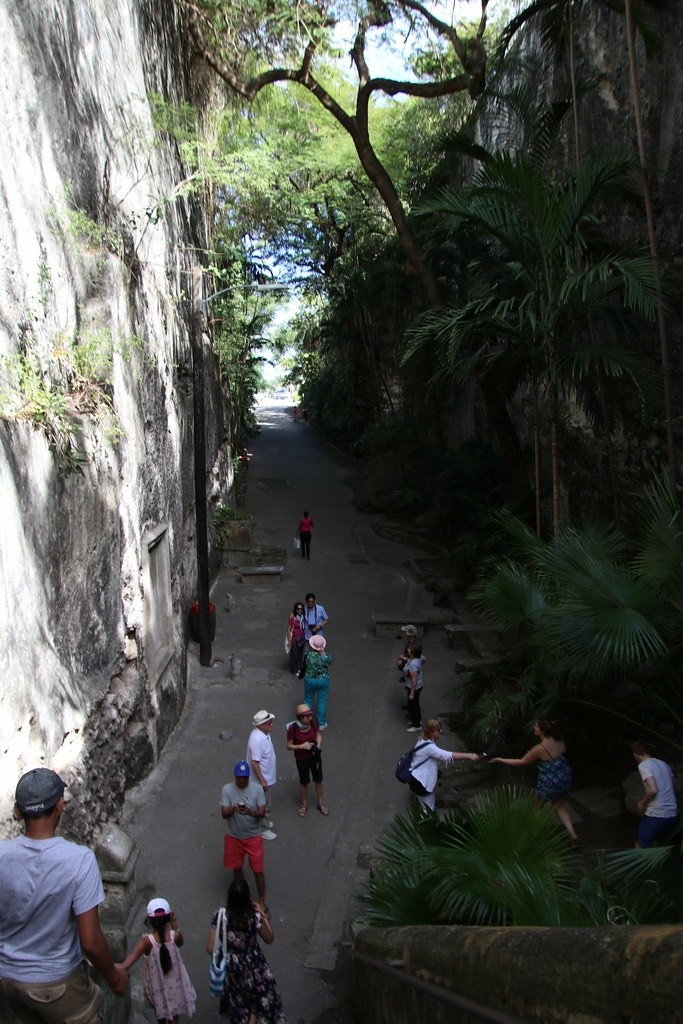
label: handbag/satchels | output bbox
[284,630,293,655]
[208,908,228,994]
[293,537,300,549]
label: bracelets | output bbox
[320,626,321,629]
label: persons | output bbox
[246,710,277,840]
[206,878,286,1024]
[488,716,582,849]
[296,511,315,560]
[115,897,198,1024]
[0,768,130,1024]
[303,635,332,730]
[219,760,271,920]
[631,740,678,849]
[397,636,427,732]
[408,719,480,815]
[287,593,329,676]
[287,704,330,816]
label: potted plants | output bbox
[212,501,253,551]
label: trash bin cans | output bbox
[192,599,216,643]
[301,409,307,417]
[240,455,248,467]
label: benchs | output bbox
[371,611,429,640]
[238,565,285,584]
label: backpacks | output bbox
[395,741,434,783]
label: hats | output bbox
[295,704,314,716]
[16,768,69,814]
[147,898,171,917]
[309,635,326,651]
[400,624,418,635]
[234,759,251,778]
[253,710,276,725]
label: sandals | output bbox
[298,803,309,817]
[315,803,330,816]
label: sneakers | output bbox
[268,821,273,828]
[261,829,277,840]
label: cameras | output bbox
[310,743,322,754]
[309,624,317,635]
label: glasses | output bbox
[308,599,316,604]
[296,607,304,611]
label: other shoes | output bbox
[301,553,305,558]
[318,723,329,731]
[306,555,310,560]
[261,905,270,920]
[294,668,301,676]
[401,704,423,733]
